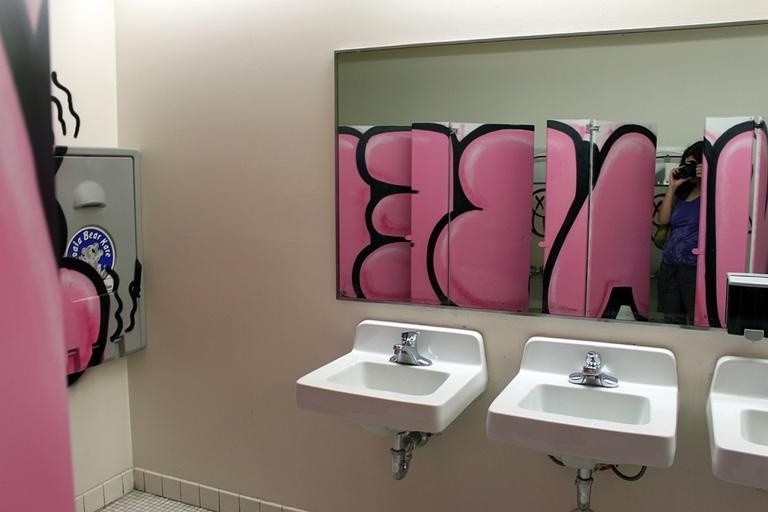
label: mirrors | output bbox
[326,22,765,338]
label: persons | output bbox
[658,140,705,325]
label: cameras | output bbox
[673,160,696,181]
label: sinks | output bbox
[485,335,680,469]
[295,319,489,435]
[703,355,768,491]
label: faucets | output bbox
[566,350,619,388]
[389,330,432,366]
